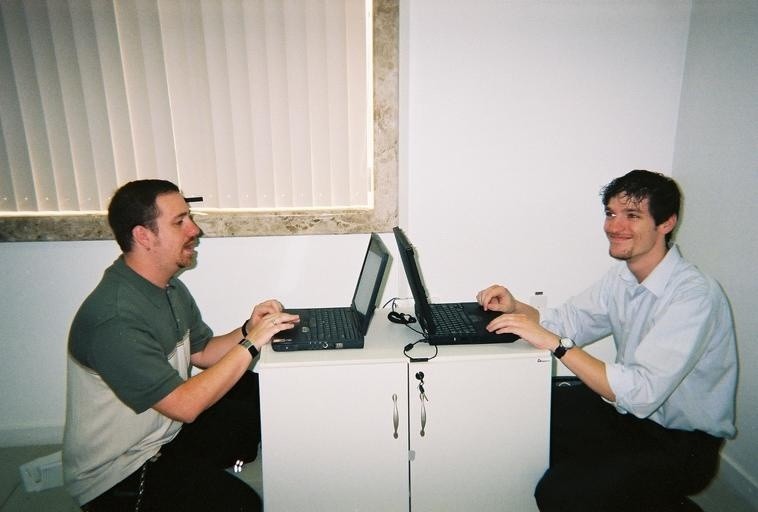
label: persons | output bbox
[60,178,302,512]
[475,169,742,510]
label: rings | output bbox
[270,319,279,327]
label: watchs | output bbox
[551,336,578,359]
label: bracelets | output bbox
[237,338,260,360]
[241,319,252,337]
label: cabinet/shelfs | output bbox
[258,308,552,512]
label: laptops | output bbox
[271,232,393,351]
[393,227,520,345]
